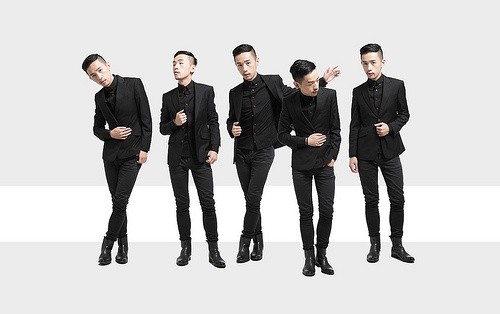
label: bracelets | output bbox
[305,137,307,146]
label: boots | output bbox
[389,236,415,263]
[303,250,315,276]
[177,239,191,266]
[206,240,225,268]
[251,233,263,261]
[99,236,114,265]
[116,234,128,264]
[367,235,380,263]
[237,237,251,263]
[315,244,334,275]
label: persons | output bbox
[159,51,227,269]
[82,54,152,267]
[278,60,342,277]
[226,44,341,263]
[348,43,415,264]
